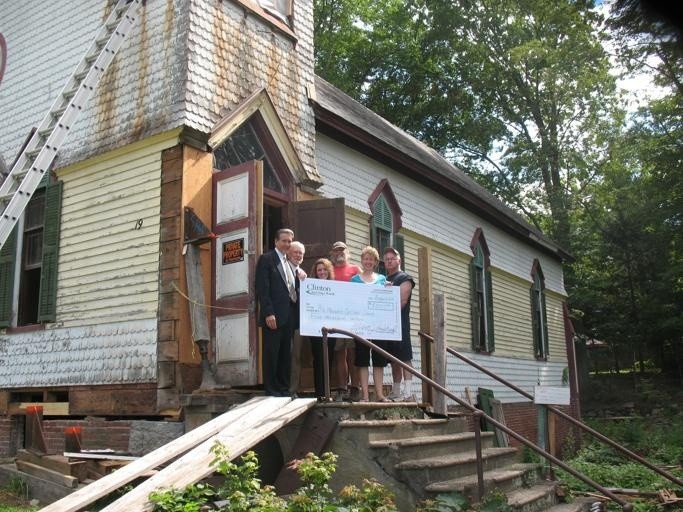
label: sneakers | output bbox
[334,386,416,402]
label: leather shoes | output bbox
[265,390,294,397]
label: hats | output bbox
[332,241,347,250]
[384,247,399,255]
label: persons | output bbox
[307,257,337,403]
[254,227,302,398]
[353,246,394,403]
[326,240,364,402]
[378,247,417,403]
[284,240,308,398]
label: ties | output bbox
[283,257,298,304]
